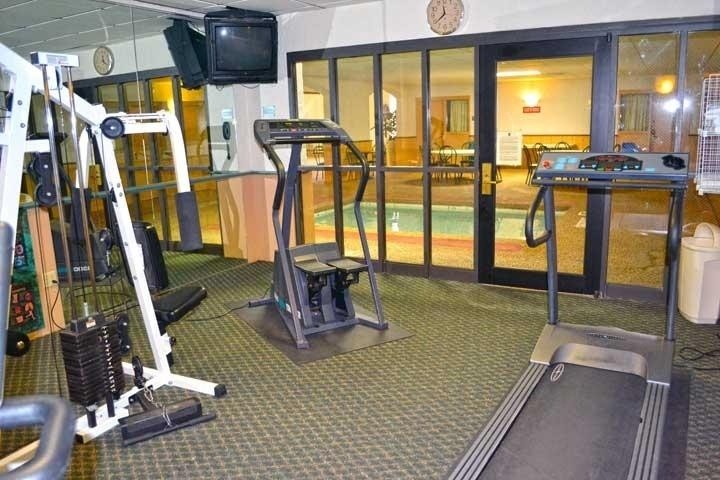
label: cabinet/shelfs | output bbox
[693,73,720,196]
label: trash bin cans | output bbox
[679,222,720,324]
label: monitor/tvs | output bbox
[203,9,277,84]
[162,20,207,89]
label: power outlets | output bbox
[46,269,58,286]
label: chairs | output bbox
[522,140,621,188]
[312,145,325,180]
[419,142,502,184]
[345,146,386,181]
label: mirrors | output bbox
[0,0,250,330]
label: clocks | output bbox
[93,45,114,76]
[426,0,465,35]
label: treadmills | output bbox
[443,153,690,480]
